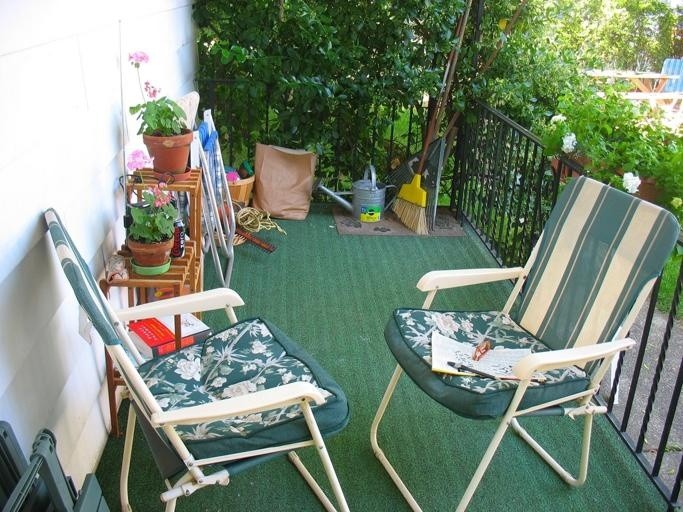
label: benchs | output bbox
[625,94,683,112]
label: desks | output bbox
[588,69,683,92]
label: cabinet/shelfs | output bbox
[99,168,202,438]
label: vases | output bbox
[126,234,174,267]
[143,129,192,174]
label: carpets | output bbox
[333,207,466,237]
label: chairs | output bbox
[44,207,351,512]
[370,176,680,512]
[654,59,683,108]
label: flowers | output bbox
[127,48,187,136]
[130,182,177,241]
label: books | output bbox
[126,312,211,359]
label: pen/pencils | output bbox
[447,362,498,380]
